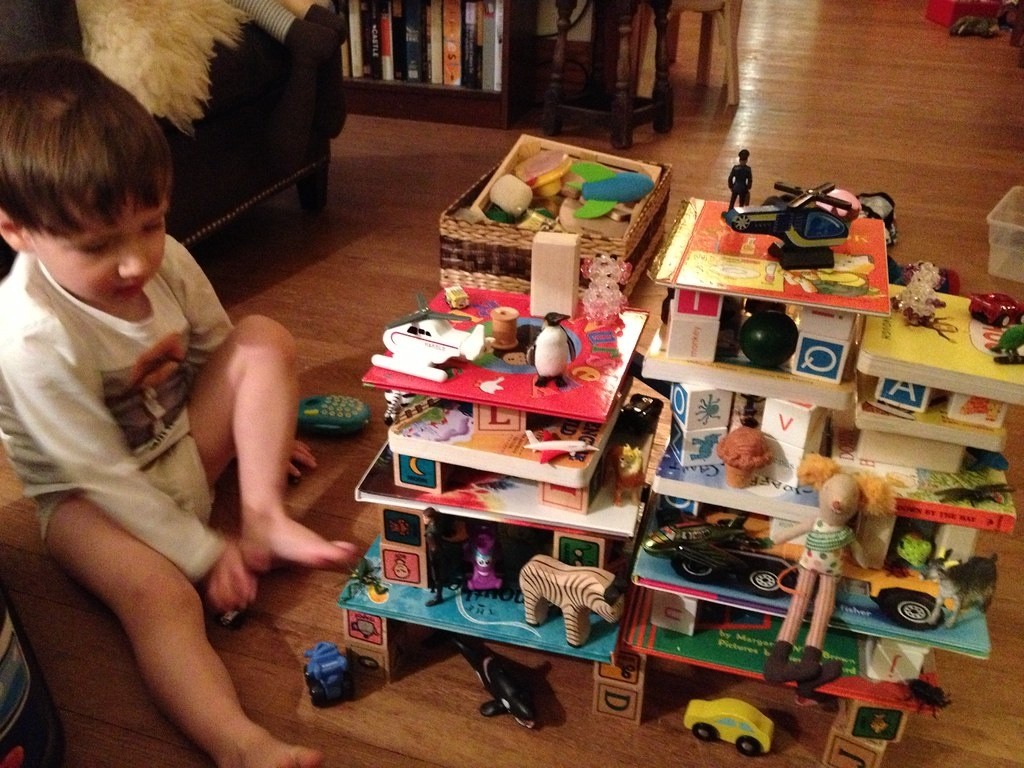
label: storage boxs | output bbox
[986,185,1024,286]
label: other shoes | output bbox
[997,11,1016,32]
[950,16,998,38]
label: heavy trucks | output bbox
[671,512,958,631]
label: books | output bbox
[331,0,495,92]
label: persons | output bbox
[762,452,896,697]
[422,507,463,606]
[728,149,752,211]
[0,57,357,768]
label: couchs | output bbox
[0,0,350,281]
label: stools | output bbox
[544,0,674,149]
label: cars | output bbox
[967,292,1023,326]
[683,698,775,757]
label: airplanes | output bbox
[641,514,748,561]
[523,430,599,464]
[455,637,548,728]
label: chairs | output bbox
[635,0,745,107]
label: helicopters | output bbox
[720,180,851,267]
[371,293,495,383]
